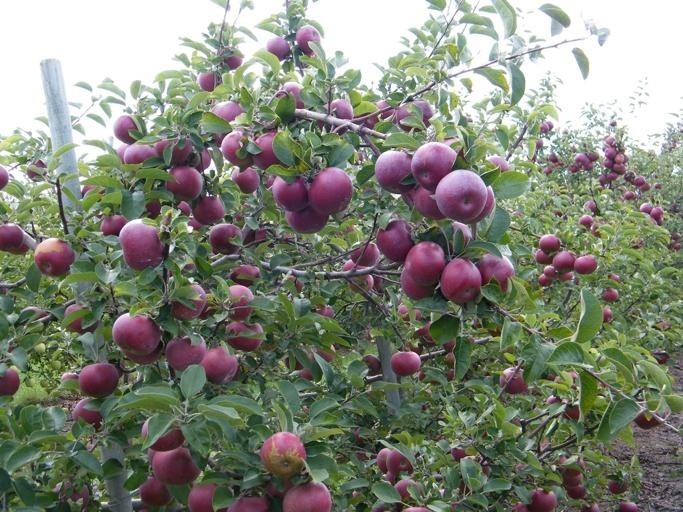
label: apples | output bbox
[0,26,683,512]
